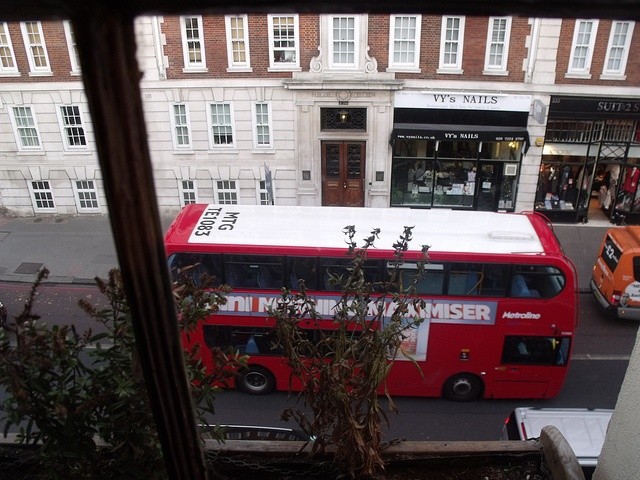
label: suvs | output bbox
[590,225,640,320]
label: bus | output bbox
[164,203,580,402]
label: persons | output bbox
[461,166,477,204]
[511,340,553,363]
[415,165,425,184]
[575,167,587,191]
[623,167,639,193]
[189,257,224,289]
[559,166,571,200]
[235,334,249,353]
[544,162,558,195]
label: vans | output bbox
[502,406,613,479]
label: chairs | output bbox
[257,268,274,289]
[225,265,247,287]
[290,271,298,290]
[516,275,541,298]
